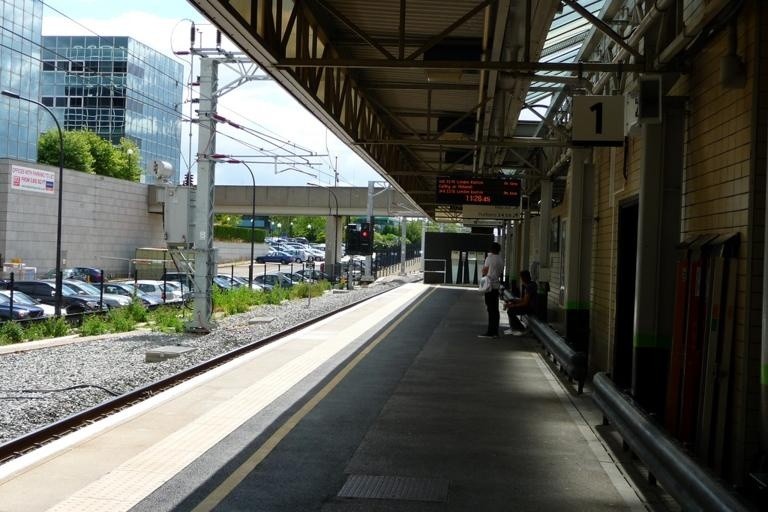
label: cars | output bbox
[257,242,347,264]
[336,255,378,280]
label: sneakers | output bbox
[478,332,500,339]
[503,328,514,335]
[513,330,528,337]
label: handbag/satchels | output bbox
[478,276,493,294]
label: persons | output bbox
[503,271,539,336]
[478,243,505,339]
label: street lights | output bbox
[306,181,339,282]
[265,218,313,243]
[1,88,64,317]
[207,153,256,292]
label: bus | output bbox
[230,214,270,228]
[355,217,402,236]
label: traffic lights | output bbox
[360,222,370,252]
